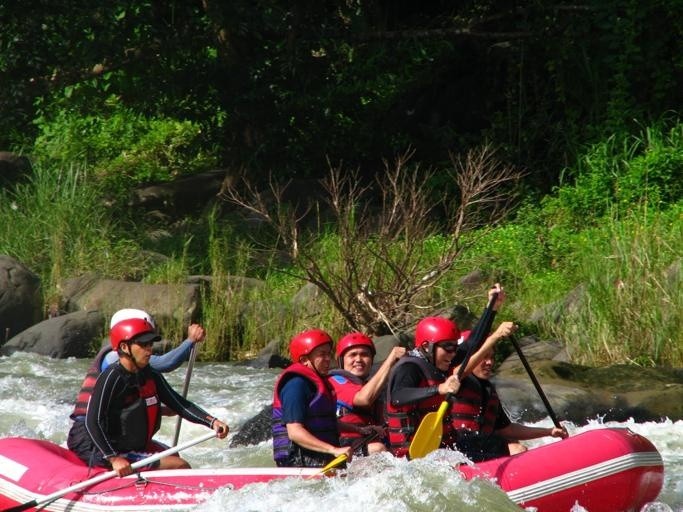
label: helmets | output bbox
[110,308,154,329]
[459,329,497,354]
[290,329,333,363]
[415,317,460,347]
[336,333,376,357]
[110,318,153,351]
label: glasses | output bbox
[437,343,459,353]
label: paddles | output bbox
[0,430,221,512]
[308,421,388,481]
[409,291,498,461]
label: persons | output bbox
[66,308,207,464]
[269,330,353,468]
[328,334,407,455]
[452,320,568,465]
[85,319,228,477]
[385,281,508,468]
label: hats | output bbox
[135,333,162,343]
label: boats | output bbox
[0,425,664,512]
